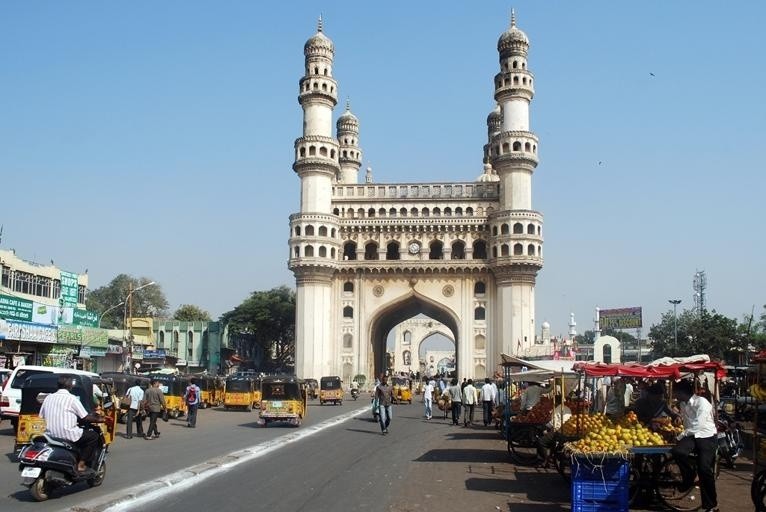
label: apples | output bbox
[565,411,684,452]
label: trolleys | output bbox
[499,413,705,511]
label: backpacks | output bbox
[188,388,196,403]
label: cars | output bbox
[722,395,766,413]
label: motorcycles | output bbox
[320,375,344,405]
[391,376,413,404]
[13,375,117,457]
[370,395,380,423]
[434,377,460,400]
[716,399,745,468]
[472,379,497,407]
[15,400,115,503]
[350,385,361,400]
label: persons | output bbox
[143,379,167,440]
[422,376,504,427]
[2,371,12,391]
[373,373,404,435]
[184,378,200,428]
[125,379,146,439]
[38,376,105,474]
[350,380,361,396]
[606,381,678,479]
[92,382,108,408]
[519,382,554,413]
[309,380,316,400]
[545,395,571,465]
[674,379,719,512]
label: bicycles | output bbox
[439,394,455,420]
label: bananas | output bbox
[750,383,766,400]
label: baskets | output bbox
[570,498,631,512]
[571,479,630,502]
[570,455,631,483]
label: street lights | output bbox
[122,280,156,373]
[666,299,683,348]
[693,270,707,314]
[97,302,124,328]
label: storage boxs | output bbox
[567,454,630,512]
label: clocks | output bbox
[408,243,421,254]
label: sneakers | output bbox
[383,428,392,435]
[124,434,159,440]
[428,416,492,428]
